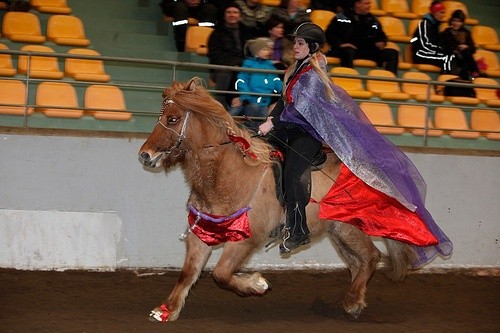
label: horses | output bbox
[138,76,420,323]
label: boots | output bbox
[279,204,310,249]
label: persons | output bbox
[441,9,480,77]
[0,0,31,34]
[158,0,348,116]
[258,22,327,253]
[409,0,471,80]
[325,0,399,75]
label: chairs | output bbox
[0,0,131,121]
[163,0,500,141]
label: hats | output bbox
[290,22,325,43]
[244,37,275,55]
